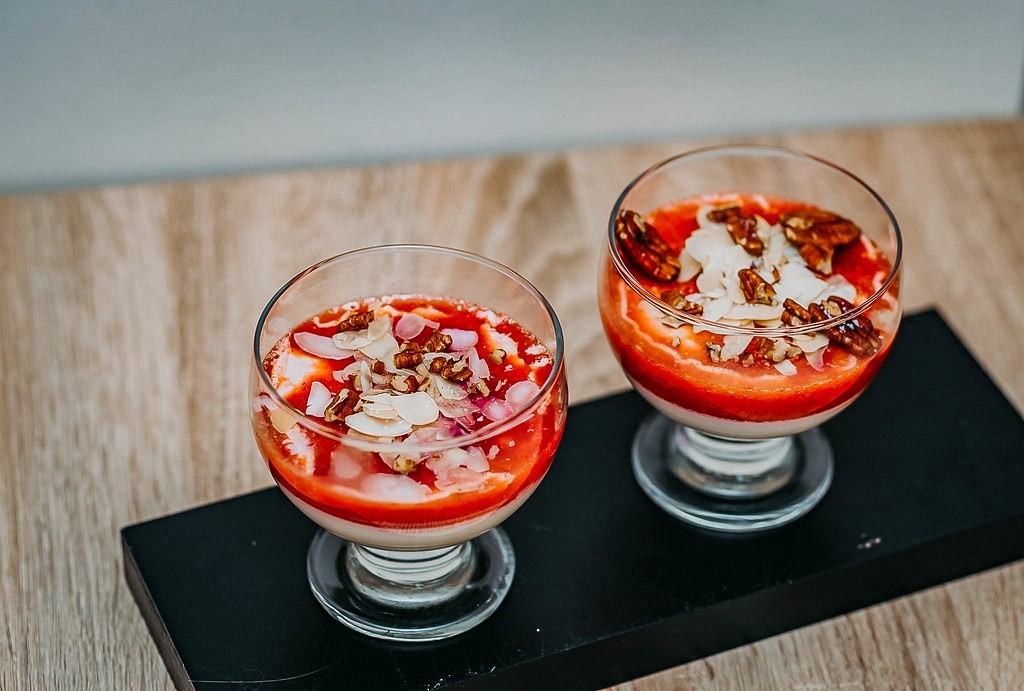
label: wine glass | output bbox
[249,244,569,641]
[597,143,902,533]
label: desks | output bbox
[0,117,1024,691]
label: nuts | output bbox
[323,310,507,422]
[615,202,882,357]
